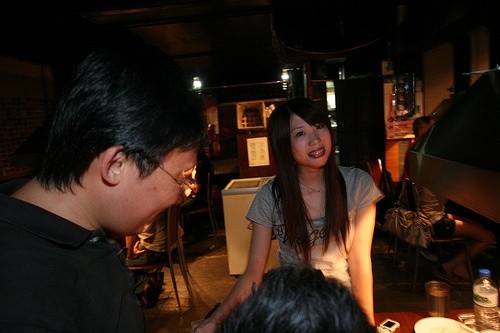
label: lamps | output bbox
[192,75,203,95]
[280,68,290,90]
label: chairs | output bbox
[124,202,194,316]
[185,168,221,238]
[360,157,474,297]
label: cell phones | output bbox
[378,319,400,333]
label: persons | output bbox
[122,212,176,310]
[0,38,208,333]
[402,115,495,286]
[191,96,376,333]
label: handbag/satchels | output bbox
[386,178,434,248]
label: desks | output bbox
[373,308,500,333]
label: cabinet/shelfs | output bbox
[221,176,282,281]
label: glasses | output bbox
[155,163,197,206]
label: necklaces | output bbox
[300,181,324,192]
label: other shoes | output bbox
[141,272,164,308]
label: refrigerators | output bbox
[222,175,282,276]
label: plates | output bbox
[413,316,479,333]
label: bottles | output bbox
[472,269,500,333]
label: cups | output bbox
[425,281,450,318]
